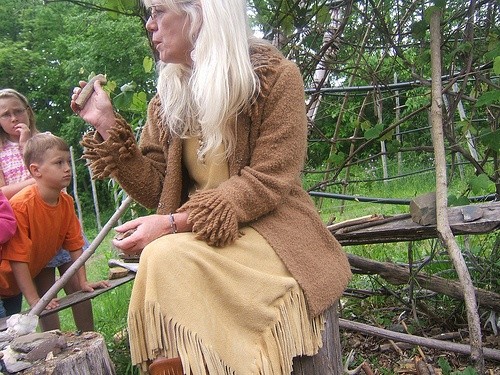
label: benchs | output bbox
[0,271,138,332]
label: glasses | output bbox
[0,105,30,118]
[148,1,193,19]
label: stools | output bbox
[291,297,345,375]
[0,330,118,375]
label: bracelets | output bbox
[169,213,178,234]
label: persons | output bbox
[0,87,95,333]
[0,131,110,316]
[70,0,354,375]
[0,189,18,318]
